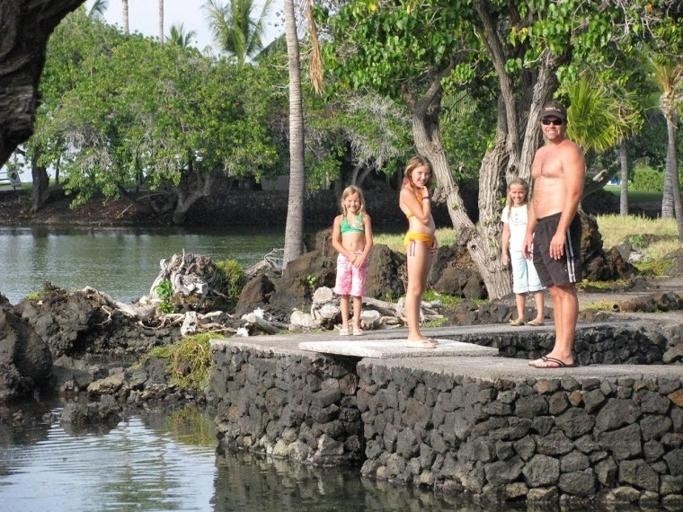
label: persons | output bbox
[398,154,439,348]
[330,184,373,336]
[522,99,586,369]
[497,177,547,326]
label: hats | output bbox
[539,100,567,121]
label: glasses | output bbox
[542,118,562,125]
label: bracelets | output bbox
[422,196,430,200]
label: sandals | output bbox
[528,319,545,326]
[406,338,438,348]
[340,327,363,336]
[509,318,527,326]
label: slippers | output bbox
[528,354,578,368]
